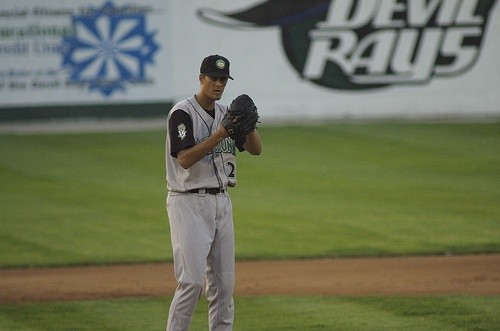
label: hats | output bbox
[200,55,235,80]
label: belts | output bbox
[188,188,225,195]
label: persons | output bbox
[165,53,262,331]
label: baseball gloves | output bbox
[219,94,261,139]
